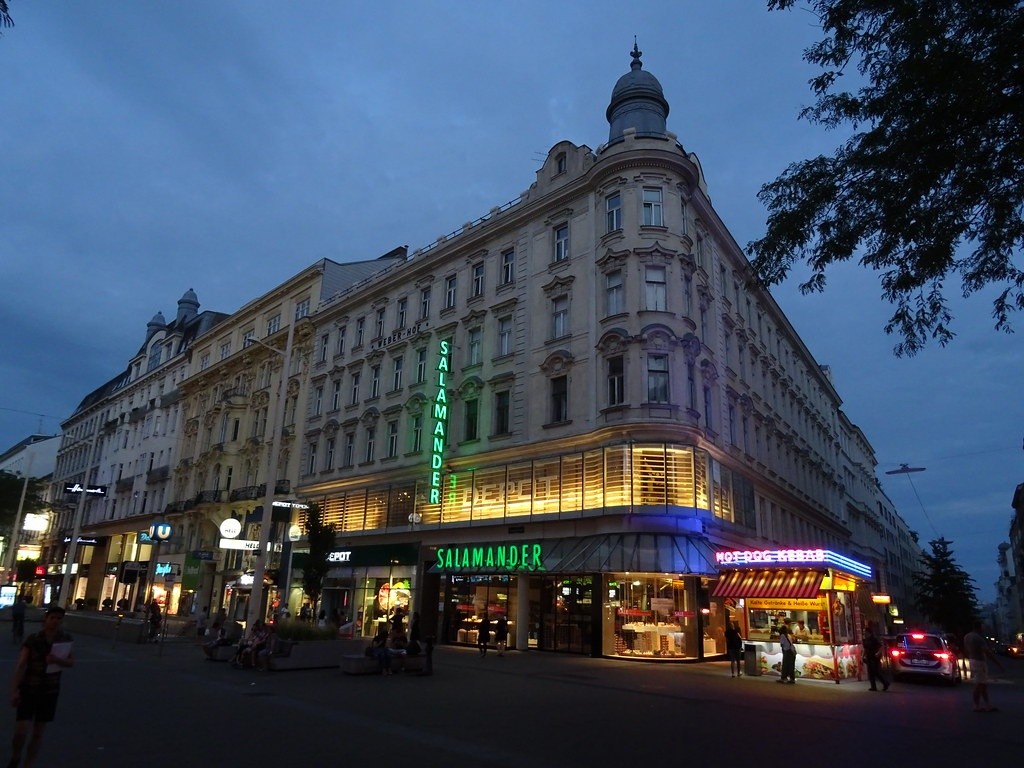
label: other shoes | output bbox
[882,682,890,691]
[868,688,877,692]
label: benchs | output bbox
[217,637,300,670]
[340,652,427,675]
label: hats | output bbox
[865,628,873,634]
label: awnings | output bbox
[710,571,825,599]
[858,583,876,610]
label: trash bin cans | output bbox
[744,644,762,676]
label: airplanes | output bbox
[885,464,926,475]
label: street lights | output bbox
[58,434,97,609]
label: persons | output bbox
[11,594,28,645]
[492,615,509,657]
[862,627,891,692]
[963,620,1005,712]
[6,606,75,768]
[478,612,490,657]
[775,618,811,684]
[149,599,424,678]
[724,621,742,677]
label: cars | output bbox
[890,633,961,687]
